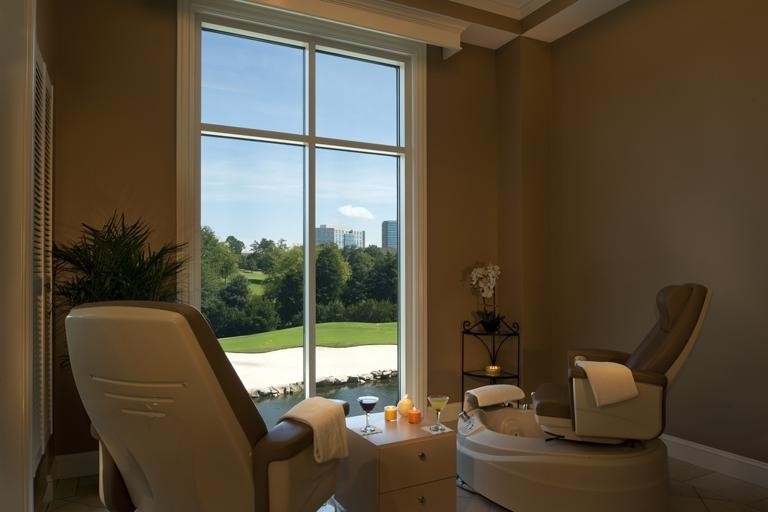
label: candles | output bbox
[384,394,421,424]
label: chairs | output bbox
[65,301,349,511]
[528,282,712,446]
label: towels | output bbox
[575,358,639,408]
[275,395,348,463]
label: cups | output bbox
[383,405,397,422]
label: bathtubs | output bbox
[455,405,671,512]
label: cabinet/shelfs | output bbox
[336,410,458,512]
[462,318,520,409]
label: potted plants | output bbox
[478,312,505,332]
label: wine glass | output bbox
[427,394,450,432]
[356,394,380,434]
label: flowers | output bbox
[470,263,503,311]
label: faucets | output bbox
[505,400,518,409]
